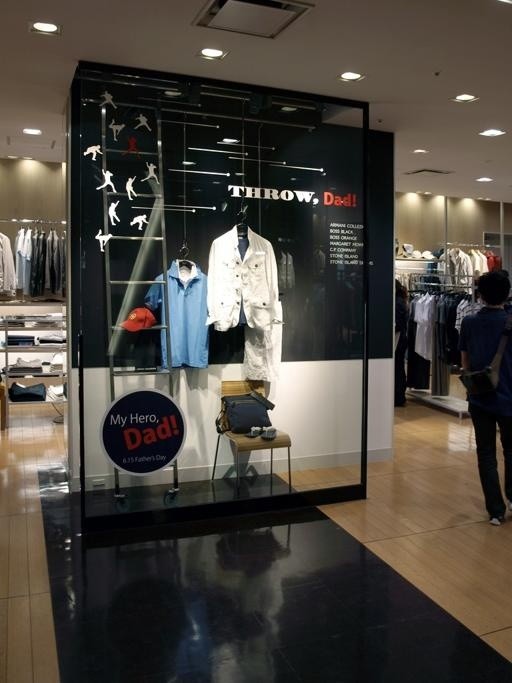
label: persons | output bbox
[396,280,410,407]
[459,271,512,526]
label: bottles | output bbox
[118,307,156,332]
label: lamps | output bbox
[211,381,292,496]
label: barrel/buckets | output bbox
[246,426,276,440]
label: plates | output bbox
[2,305,67,429]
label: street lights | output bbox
[220,393,273,433]
[461,368,499,395]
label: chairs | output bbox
[507,501,512,511]
[489,513,504,525]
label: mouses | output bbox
[235,210,250,240]
[175,245,194,274]
